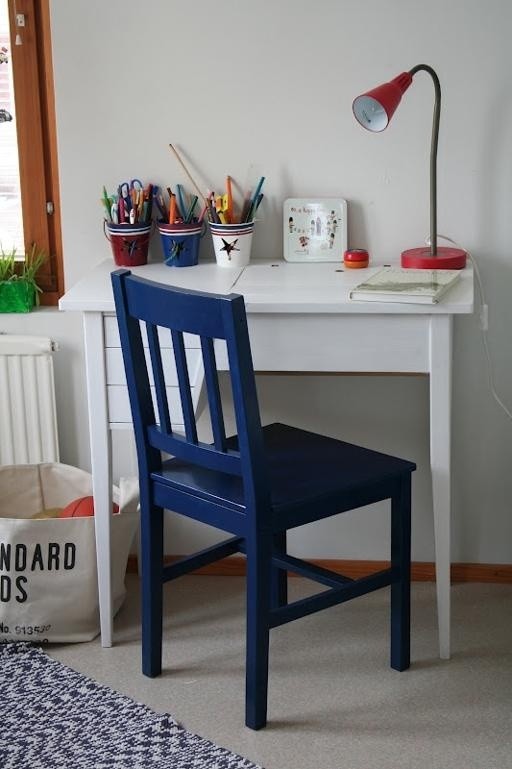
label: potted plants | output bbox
[0,243,56,315]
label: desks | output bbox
[54,262,473,660]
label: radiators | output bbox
[0,334,61,464]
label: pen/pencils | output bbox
[154,184,212,225]
[206,176,265,223]
[102,184,154,225]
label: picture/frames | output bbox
[282,197,349,262]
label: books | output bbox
[348,265,463,307]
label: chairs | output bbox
[106,266,420,729]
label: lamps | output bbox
[354,64,471,273]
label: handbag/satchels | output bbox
[0,462,141,644]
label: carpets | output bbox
[1,641,259,769]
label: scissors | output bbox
[120,179,143,211]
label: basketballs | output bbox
[60,495,119,518]
